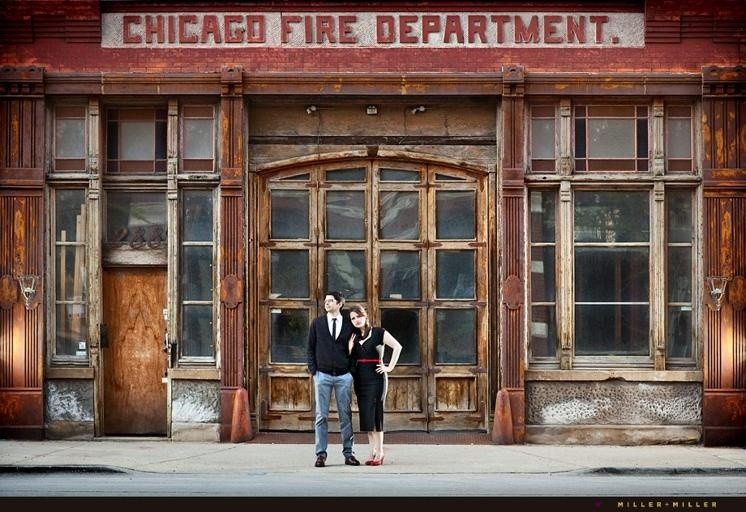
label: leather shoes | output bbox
[314,457,326,467]
[345,456,360,465]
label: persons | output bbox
[307,290,403,467]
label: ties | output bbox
[332,318,337,341]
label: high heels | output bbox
[365,455,384,465]
